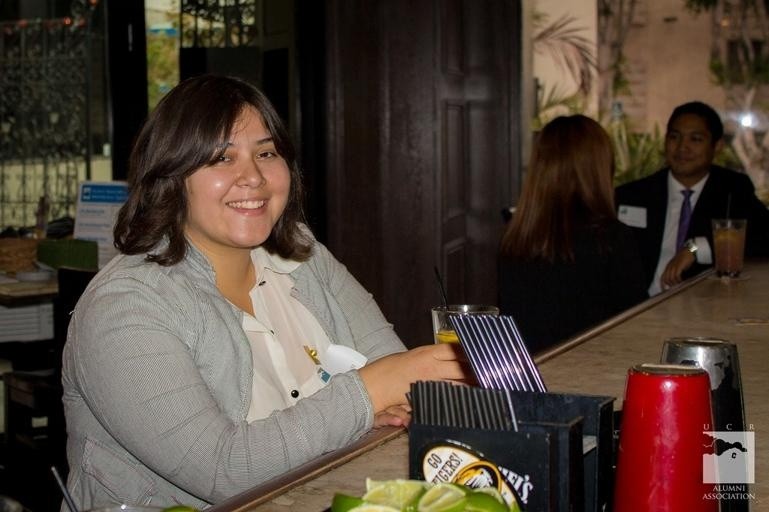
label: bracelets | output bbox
[686,242,700,254]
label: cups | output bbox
[428,303,500,382]
[711,218,748,279]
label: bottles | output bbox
[612,362,721,512]
[36,196,48,233]
[662,338,750,512]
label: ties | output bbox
[677,188,694,255]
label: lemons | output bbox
[331,476,510,511]
[163,505,197,512]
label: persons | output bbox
[614,99,769,301]
[58,71,480,512]
[492,112,653,358]
